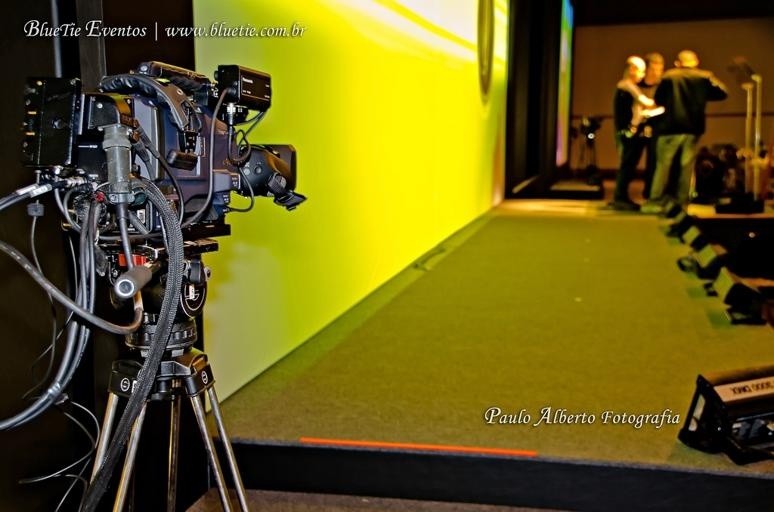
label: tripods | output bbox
[575,139,597,171]
[78,351,254,512]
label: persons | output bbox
[640,48,729,215]
[635,52,664,200]
[606,55,656,211]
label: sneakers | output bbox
[613,189,674,214]
